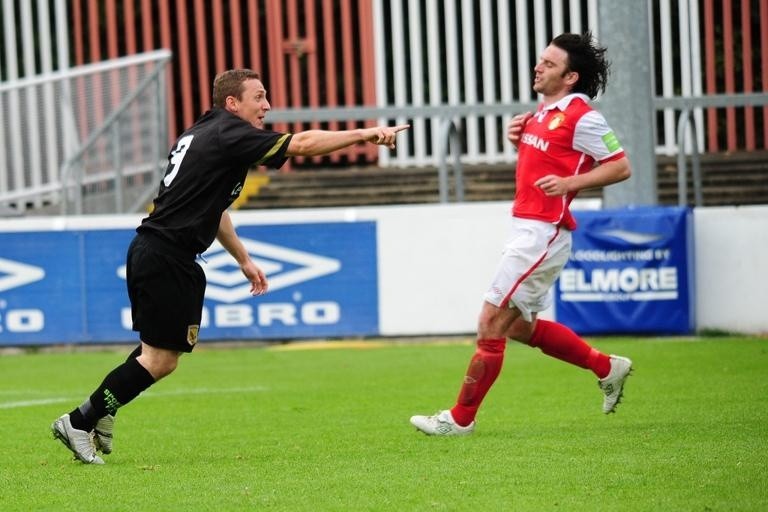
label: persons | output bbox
[51,66,412,466]
[408,29,636,437]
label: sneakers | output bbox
[409,409,476,436]
[598,355,632,413]
[50,413,115,464]
